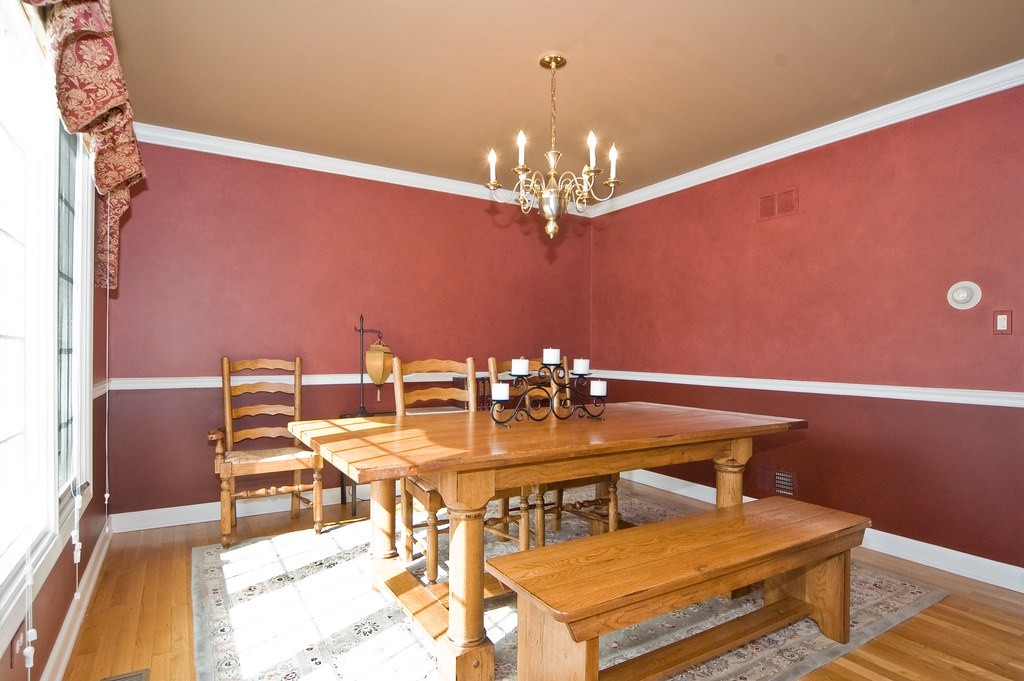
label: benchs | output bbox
[488,494,871,681]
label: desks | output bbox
[794,594,1024,681]
[290,401,810,681]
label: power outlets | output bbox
[993,311,1012,335]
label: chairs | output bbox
[392,355,530,581]
[207,355,324,548]
[486,355,619,548]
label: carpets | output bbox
[191,484,950,681]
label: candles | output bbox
[590,377,607,396]
[573,356,590,374]
[511,355,529,375]
[543,347,560,364]
[491,379,510,401]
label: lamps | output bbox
[486,53,620,240]
[352,316,393,417]
